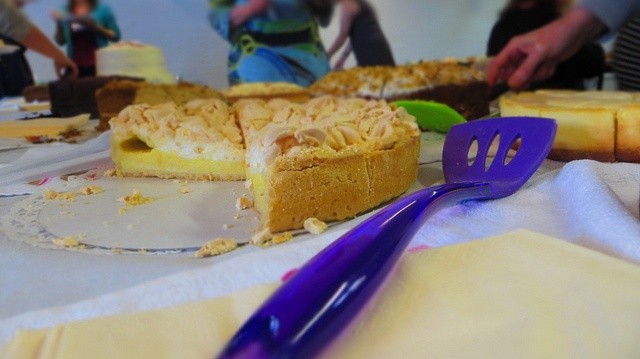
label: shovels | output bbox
[395,101,500,132]
[216,117,556,358]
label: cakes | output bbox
[46,72,145,116]
[91,38,174,87]
[24,82,51,104]
[497,85,640,165]
[101,94,424,234]
[310,54,491,124]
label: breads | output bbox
[225,74,312,107]
[90,78,226,114]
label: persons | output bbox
[488,0,605,90]
[326,0,395,71]
[0,0,79,82]
[50,0,121,78]
[207,0,334,87]
[489,0,640,90]
[0,0,35,95]
